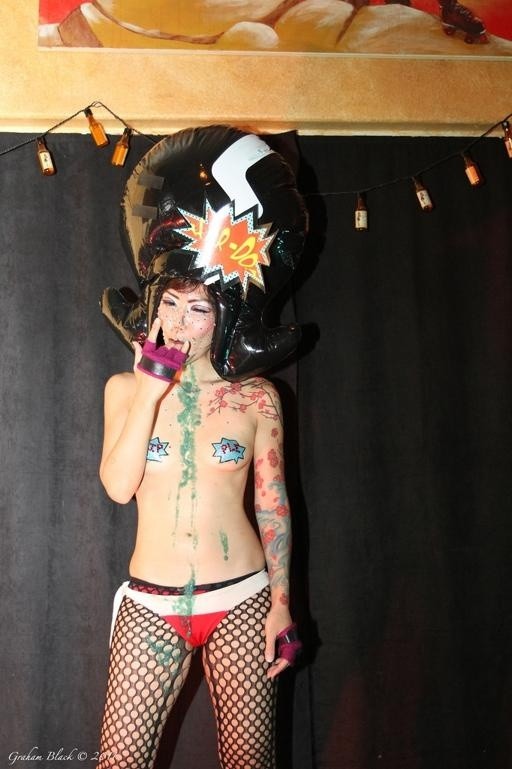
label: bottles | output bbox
[502,120,512,158]
[111,128,131,167]
[462,150,482,185]
[412,176,433,211]
[355,192,368,231]
[84,109,108,146]
[37,137,55,176]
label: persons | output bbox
[98,274,303,768]
